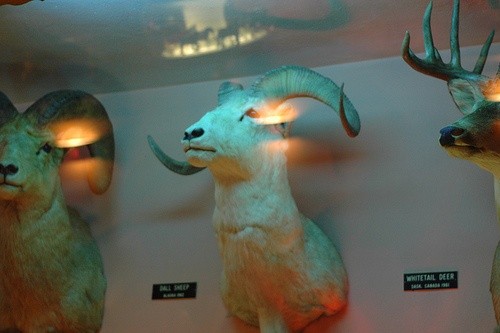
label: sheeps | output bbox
[0,88,116,333]
[146,66,361,333]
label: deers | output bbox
[401,0,500,332]
